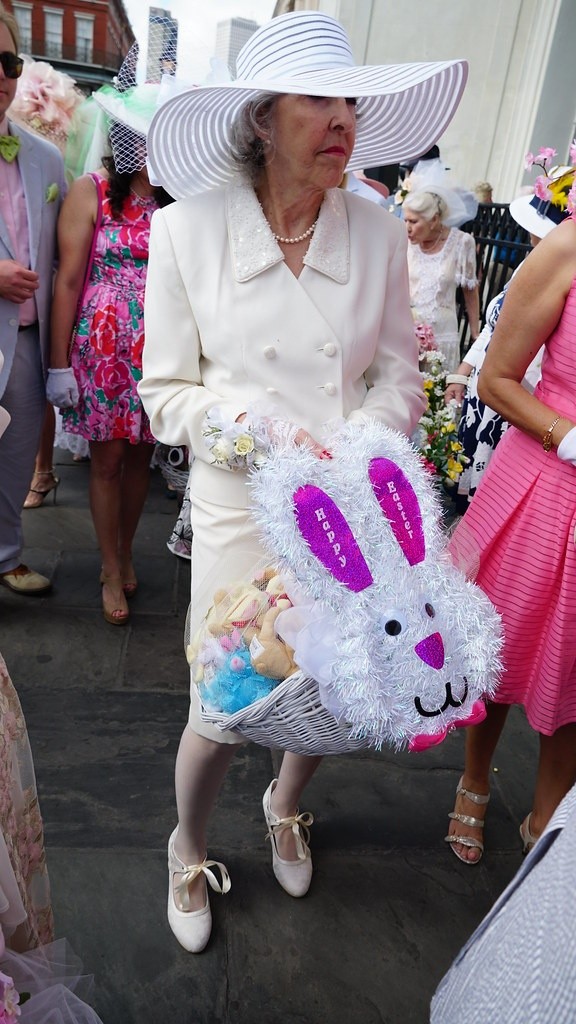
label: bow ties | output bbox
[0,135,20,162]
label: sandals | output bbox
[444,773,490,863]
[519,811,540,853]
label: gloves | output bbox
[556,425,576,465]
[46,368,79,409]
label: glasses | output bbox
[0,51,24,79]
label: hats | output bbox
[509,165,575,241]
[92,77,196,142]
[107,10,469,204]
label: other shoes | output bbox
[73,453,82,461]
[0,562,50,592]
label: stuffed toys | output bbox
[187,420,506,752]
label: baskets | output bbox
[200,574,381,756]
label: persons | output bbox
[0,9,191,620]
[0,655,105,1024]
[446,219,576,863]
[136,12,469,951]
[429,783,576,1024]
[341,146,576,528]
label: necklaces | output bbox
[420,226,443,251]
[273,218,317,244]
[131,187,156,205]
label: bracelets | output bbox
[446,374,468,385]
[542,416,563,452]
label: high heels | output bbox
[263,778,312,897]
[120,558,137,596]
[167,822,231,952]
[22,466,60,509]
[100,567,128,625]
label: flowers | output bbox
[414,321,472,484]
[201,409,256,468]
[43,179,61,203]
[527,139,576,215]
